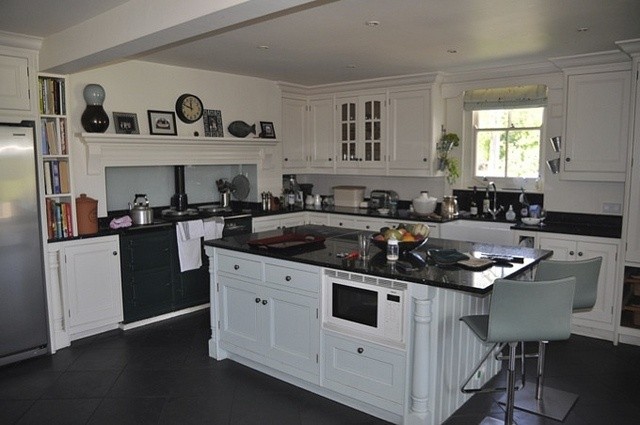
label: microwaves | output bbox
[321,267,409,350]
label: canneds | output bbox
[441,196,455,220]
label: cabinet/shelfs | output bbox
[281,97,308,169]
[330,219,356,229]
[558,65,632,182]
[64,241,124,333]
[215,248,322,386]
[309,217,330,226]
[618,58,640,338]
[355,220,384,232]
[309,99,334,168]
[538,237,618,332]
[335,93,389,170]
[36,73,78,239]
[385,223,439,240]
[0,55,31,110]
[254,216,304,233]
[388,89,447,170]
[323,335,407,407]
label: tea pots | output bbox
[128,194,153,226]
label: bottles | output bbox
[387,233,400,261]
[261,191,267,211]
[483,188,490,214]
[470,186,479,217]
[267,191,271,210]
[288,184,296,207]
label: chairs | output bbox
[500,257,601,420]
[459,277,574,425]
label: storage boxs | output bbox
[333,185,366,207]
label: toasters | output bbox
[370,189,399,209]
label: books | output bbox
[40,118,67,156]
[43,159,70,195]
[37,76,66,115]
[45,199,73,240]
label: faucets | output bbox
[484,181,505,216]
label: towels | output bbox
[110,215,132,229]
[175,220,204,273]
[203,215,225,240]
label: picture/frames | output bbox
[147,109,177,135]
[112,112,140,134]
[203,109,224,137]
[260,120,276,138]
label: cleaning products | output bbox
[518,188,530,217]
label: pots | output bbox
[75,193,99,234]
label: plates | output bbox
[427,248,470,264]
[457,257,496,269]
[369,251,427,271]
[378,208,390,214]
[369,232,429,255]
[521,217,542,225]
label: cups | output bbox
[220,193,230,208]
[357,232,371,260]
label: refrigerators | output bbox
[1,121,54,368]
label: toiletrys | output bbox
[506,205,516,220]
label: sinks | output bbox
[444,219,517,230]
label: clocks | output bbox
[175,93,204,124]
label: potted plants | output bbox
[437,133,460,151]
[440,156,460,185]
